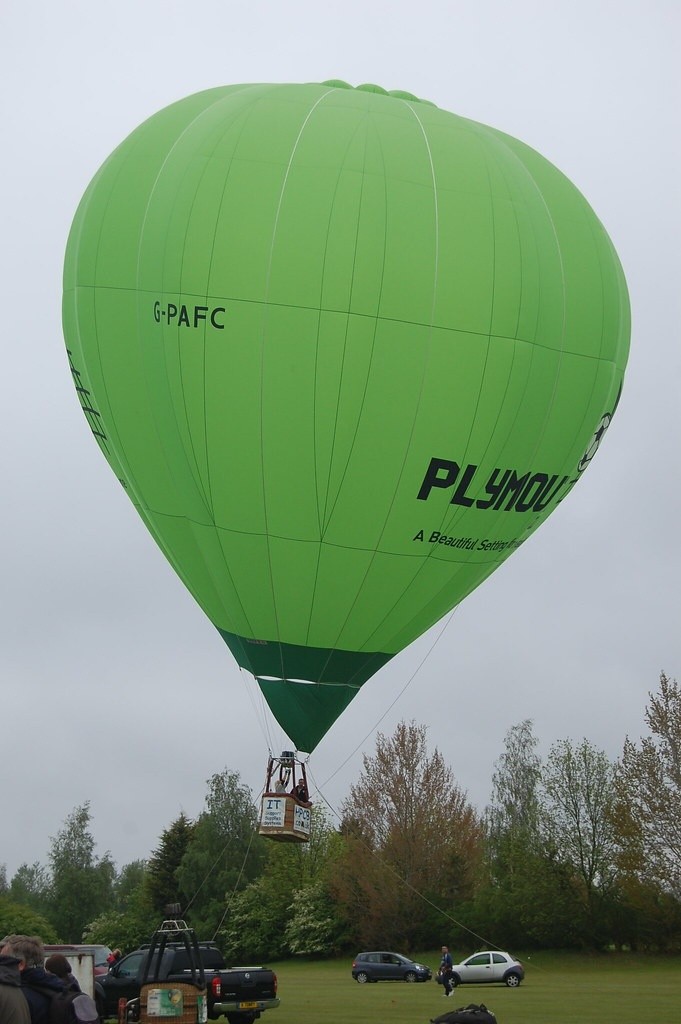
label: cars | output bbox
[436,951,525,987]
[42,944,119,977]
[351,951,433,985]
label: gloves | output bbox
[285,769,291,775]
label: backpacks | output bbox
[23,978,103,1024]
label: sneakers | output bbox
[448,990,455,997]
[443,995,448,997]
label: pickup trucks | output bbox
[94,941,281,1024]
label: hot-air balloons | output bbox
[58,79,633,844]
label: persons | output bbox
[0,935,82,1024]
[108,948,125,973]
[274,769,290,794]
[291,779,308,803]
[438,945,455,996]
